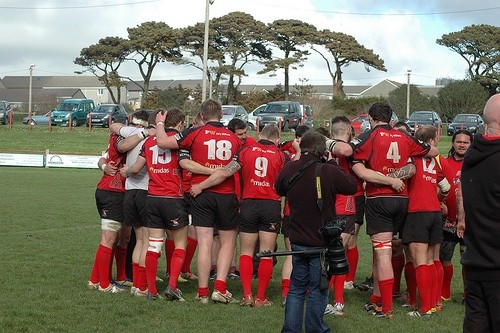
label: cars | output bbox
[85,103,129,128]
[447,113,484,136]
[22,111,57,126]
[221,105,248,126]
[349,113,367,134]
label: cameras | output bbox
[321,152,329,163]
[318,220,350,275]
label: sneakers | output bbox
[344,280,355,290]
[110,278,129,289]
[194,293,210,304]
[323,304,347,318]
[98,283,124,294]
[164,272,189,284]
[131,286,148,298]
[211,289,240,304]
[164,287,186,302]
[353,278,451,319]
[88,281,100,290]
[229,270,241,279]
[280,298,286,308]
[241,295,254,307]
[333,303,345,311]
[156,276,164,284]
[115,280,133,287]
[253,297,274,307]
[180,272,198,280]
[147,293,164,302]
[209,269,217,281]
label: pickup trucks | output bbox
[0,100,14,125]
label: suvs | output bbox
[360,112,399,132]
[404,110,442,133]
[256,101,302,132]
[51,98,95,127]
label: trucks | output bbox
[248,104,313,132]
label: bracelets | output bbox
[138,130,148,138]
[157,121,164,125]
[102,164,107,170]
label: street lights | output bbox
[407,70,412,119]
[202,0,215,104]
[28,64,36,119]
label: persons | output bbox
[273,129,358,333]
[86,99,473,318]
[459,93,500,333]
